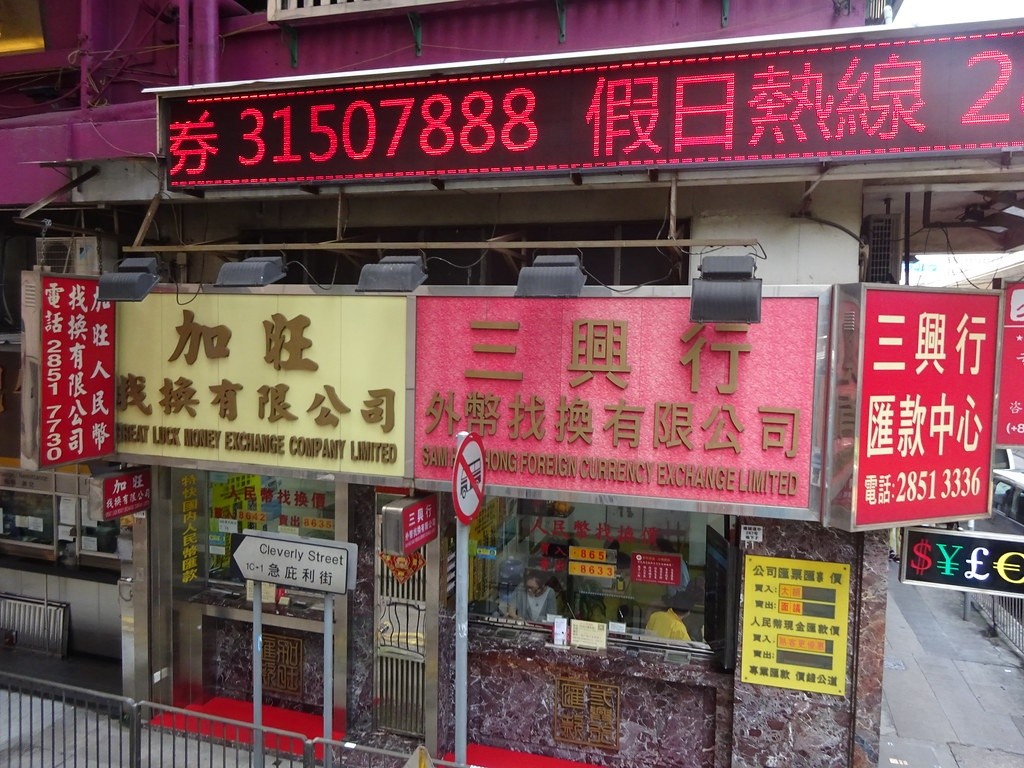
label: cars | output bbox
[980,468,1024,535]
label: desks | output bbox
[578,568,704,630]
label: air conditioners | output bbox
[860,213,903,285]
[36,236,102,276]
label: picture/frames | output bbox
[605,505,644,531]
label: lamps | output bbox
[688,245,762,323]
[1000,190,1024,218]
[95,258,160,302]
[355,255,429,292]
[515,255,588,298]
[213,256,286,288]
[967,211,1009,234]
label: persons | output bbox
[642,591,694,641]
[506,571,558,622]
[602,539,630,569]
[650,540,690,602]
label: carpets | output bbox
[149,696,345,763]
[438,742,608,768]
